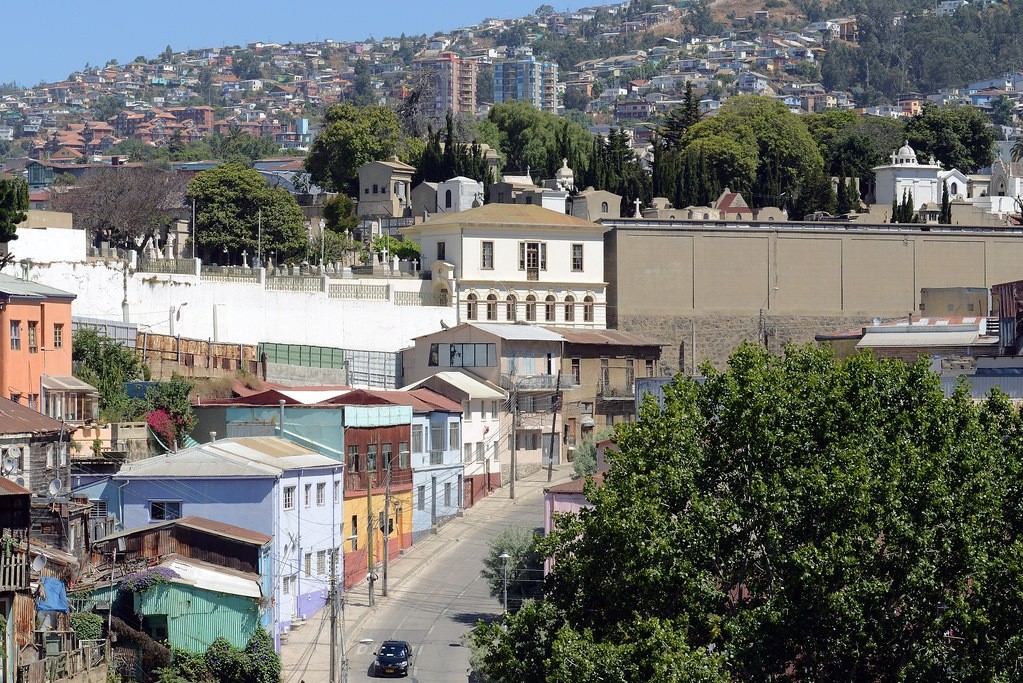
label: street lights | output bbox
[329,534,359,683]
[381,449,411,596]
[341,637,373,683]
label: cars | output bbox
[372,640,413,676]
